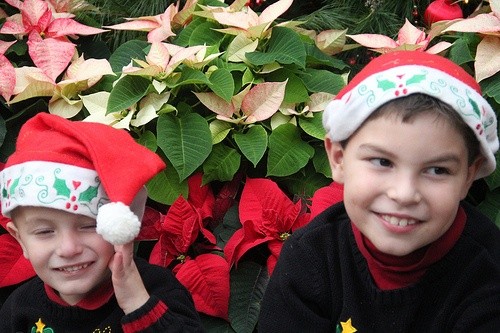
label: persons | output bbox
[249,50,499,333]
[0,112,238,333]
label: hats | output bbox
[322,50,499,181]
[0,112,166,246]
